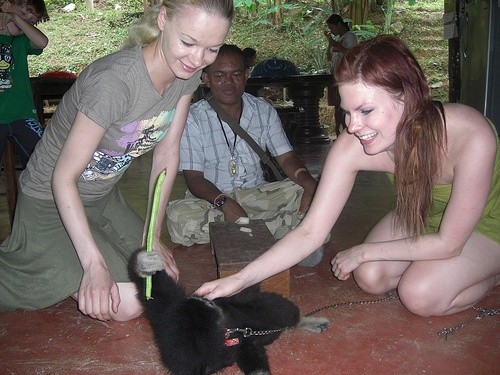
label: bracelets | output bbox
[294,167,306,178]
[212,193,224,204]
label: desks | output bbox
[195,73,335,144]
[29,77,76,127]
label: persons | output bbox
[0,0,50,162]
[191,33,500,318]
[0,0,237,323]
[164,42,333,269]
[240,47,257,75]
[325,13,359,141]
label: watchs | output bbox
[217,196,226,208]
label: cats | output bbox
[127,246,330,375]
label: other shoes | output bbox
[298,246,325,268]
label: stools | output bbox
[208,219,290,300]
